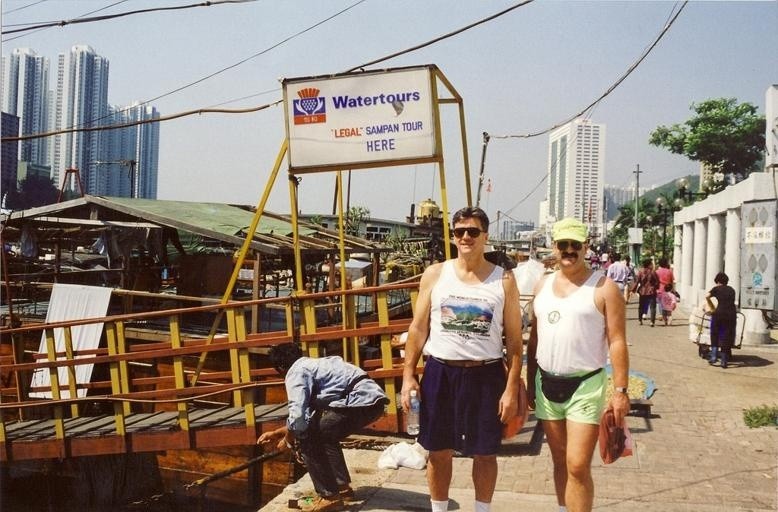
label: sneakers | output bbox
[302,487,354,511]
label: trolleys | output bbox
[687,307,747,360]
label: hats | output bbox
[551,217,590,243]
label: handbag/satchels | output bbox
[541,373,580,403]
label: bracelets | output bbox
[614,386,627,393]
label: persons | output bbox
[525,215,632,511]
[589,240,681,326]
[705,270,737,370]
[398,204,525,511]
[257,340,390,511]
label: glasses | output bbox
[556,239,583,252]
[452,226,484,239]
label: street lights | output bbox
[675,176,715,206]
[654,195,686,260]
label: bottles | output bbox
[406,390,422,436]
